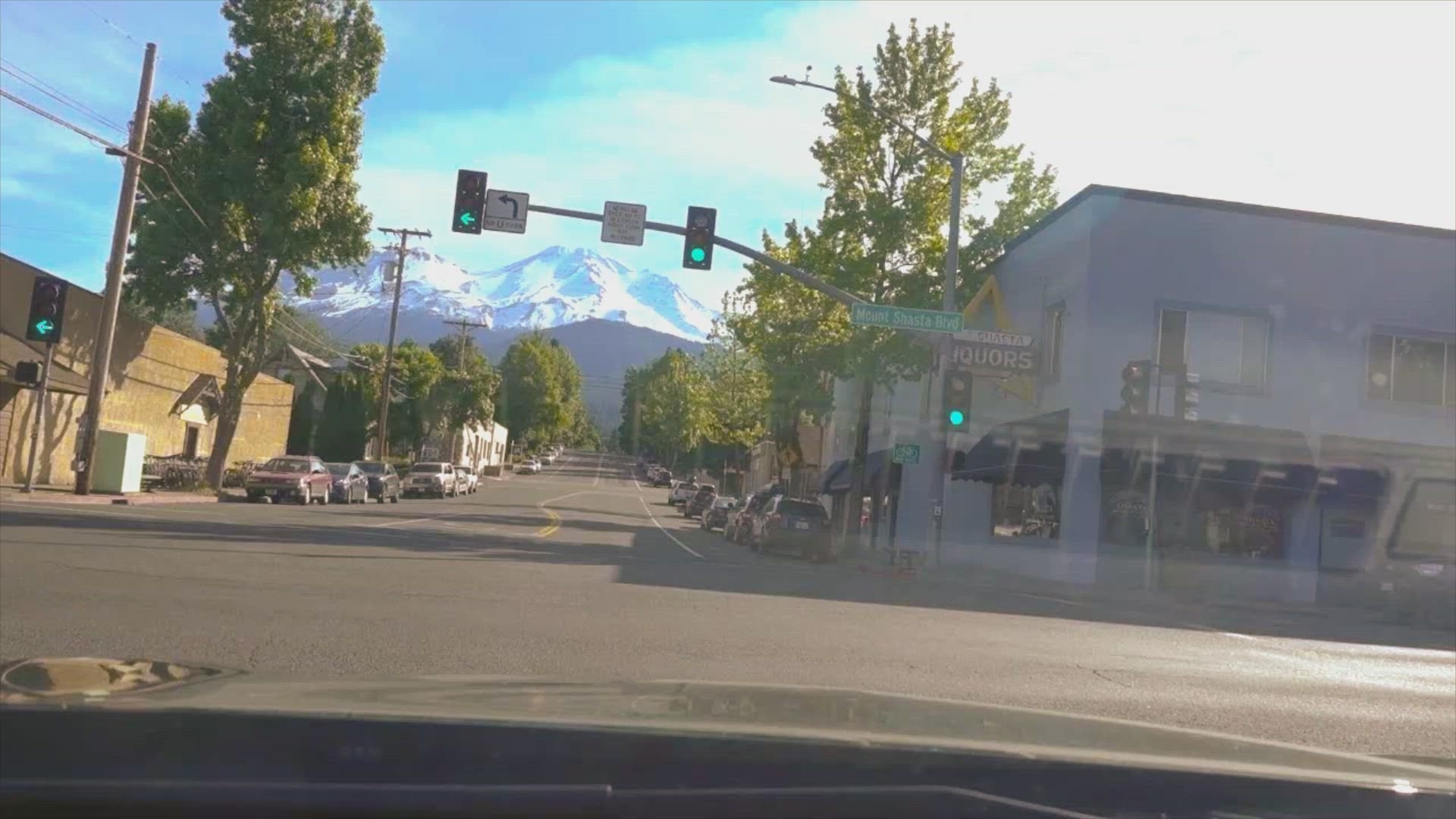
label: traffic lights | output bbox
[1173,363,1201,429]
[1118,359,1151,433]
[25,277,70,343]
[939,370,974,433]
[449,169,489,234]
[683,204,716,272]
[13,361,43,383]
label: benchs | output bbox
[886,546,920,568]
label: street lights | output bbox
[74,141,173,496]
[769,75,965,574]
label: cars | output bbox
[454,466,479,493]
[684,490,717,518]
[403,462,458,497]
[723,492,769,544]
[455,469,470,495]
[519,442,564,473]
[322,462,370,504]
[636,456,672,487]
[666,481,701,506]
[246,454,334,503]
[701,498,738,532]
[352,459,402,503]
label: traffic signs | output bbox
[482,189,531,233]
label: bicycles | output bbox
[144,452,210,491]
[223,460,266,488]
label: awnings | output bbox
[949,408,1455,500]
[805,448,901,496]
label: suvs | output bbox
[756,494,833,560]
